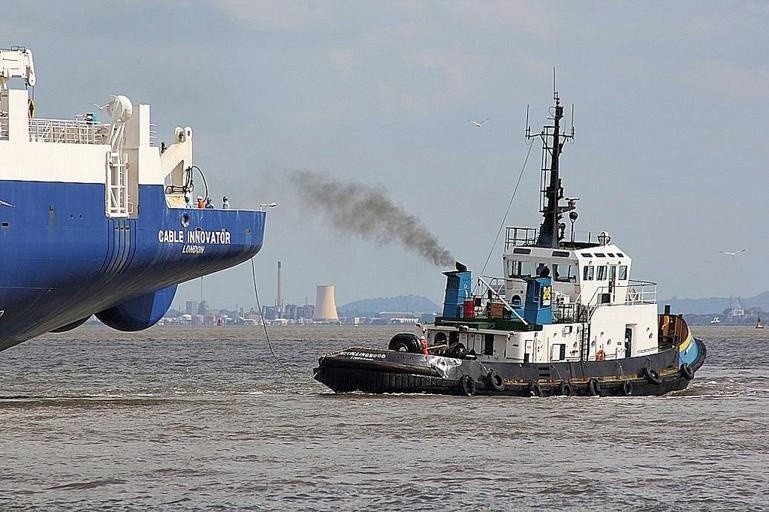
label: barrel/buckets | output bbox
[464,300,475,318]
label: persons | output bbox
[205,197,215,208]
[536,263,545,275]
[420,336,428,355]
[223,196,229,209]
[197,196,204,208]
[185,197,192,209]
[540,266,550,278]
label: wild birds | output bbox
[468,117,486,128]
[717,248,747,258]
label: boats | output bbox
[754,311,765,328]
[1,42,268,363]
[710,316,721,324]
[312,63,708,395]
